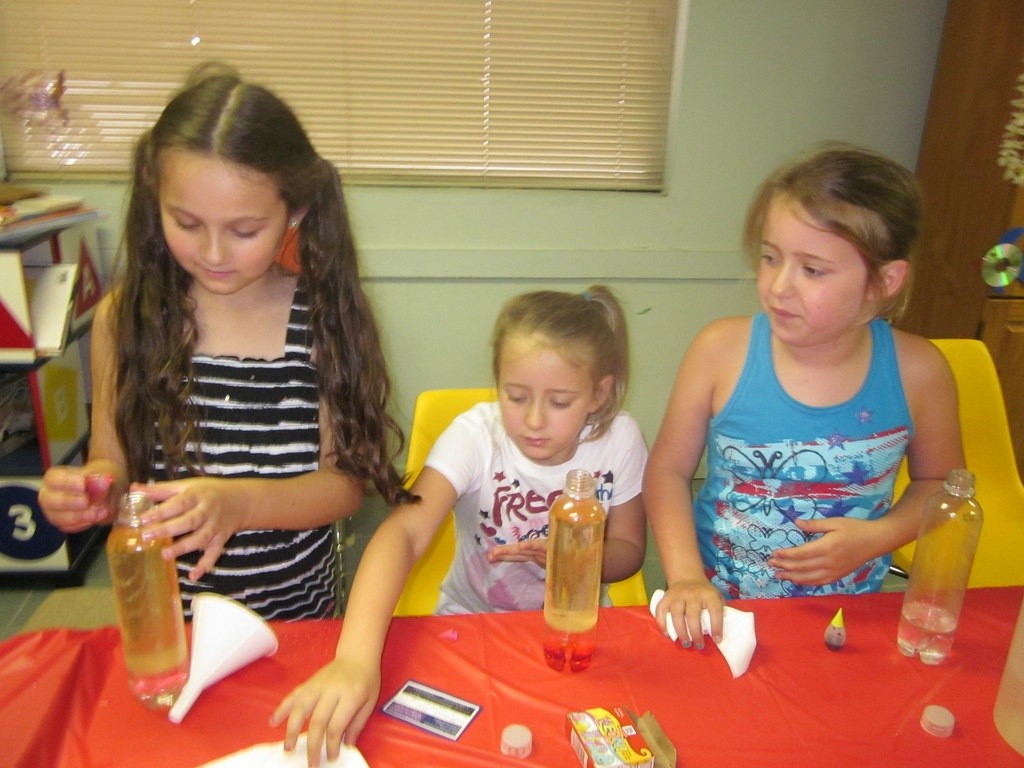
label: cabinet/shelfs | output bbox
[0,211,112,591]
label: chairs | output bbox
[890,339,1024,588]
[392,385,647,617]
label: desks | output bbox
[0,583,1024,768]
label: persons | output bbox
[39,74,420,622]
[642,148,966,651]
[269,283,649,768]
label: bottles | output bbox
[896,467,984,666]
[544,468,606,633]
[105,492,189,711]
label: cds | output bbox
[979,243,1023,289]
